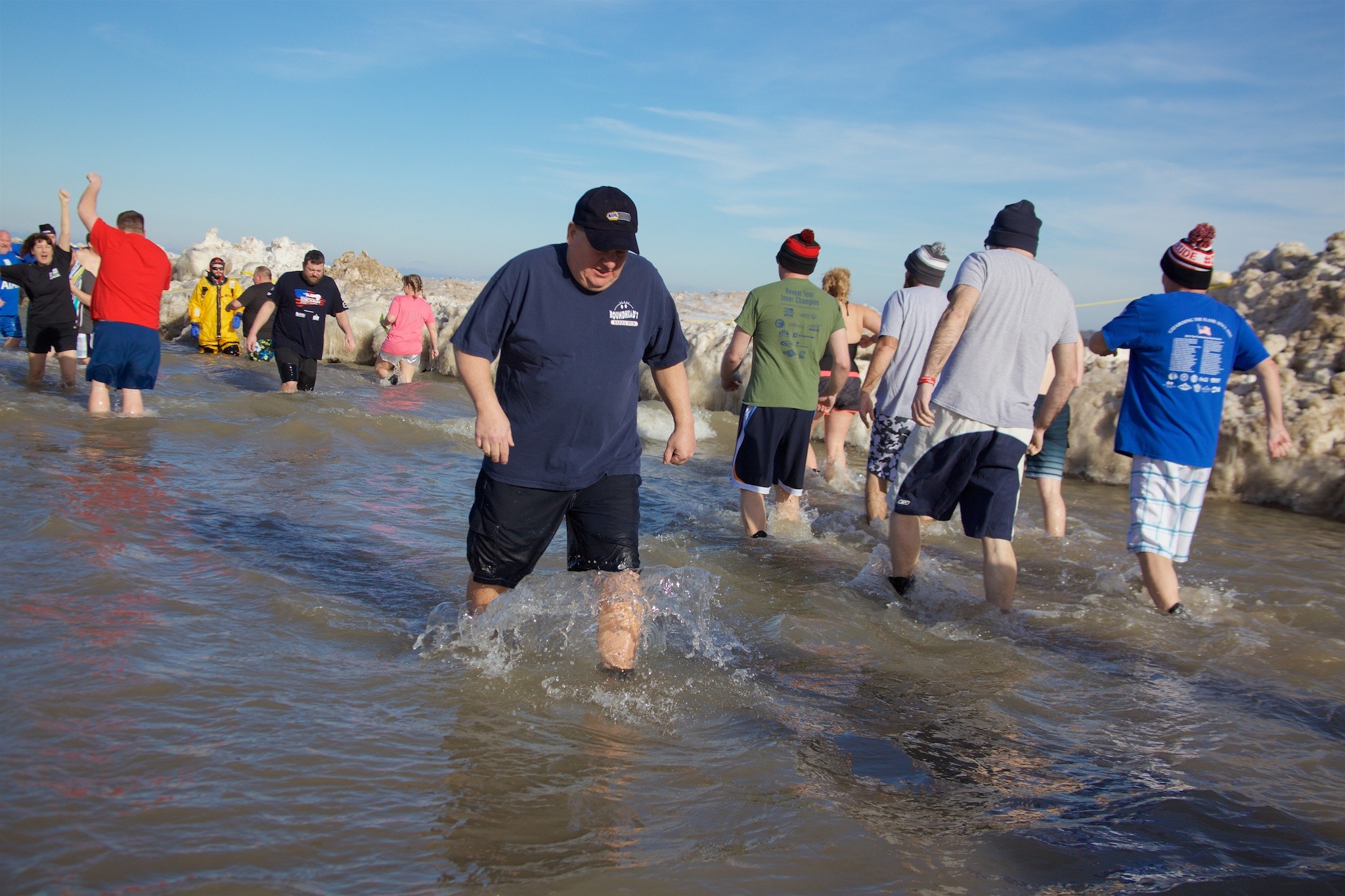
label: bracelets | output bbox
[916,377,936,386]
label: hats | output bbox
[39,223,56,235]
[210,257,225,267]
[905,241,949,288]
[1161,223,1216,290]
[777,229,820,276]
[573,186,640,255]
[984,200,1042,258]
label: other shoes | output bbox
[887,574,916,600]
[1169,603,1187,619]
[753,530,767,538]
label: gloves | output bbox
[190,323,199,339]
[232,313,243,329]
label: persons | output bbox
[884,200,1078,610]
[188,258,245,357]
[805,266,882,482]
[78,173,171,413]
[1089,221,1292,615]
[0,188,102,388]
[721,229,850,538]
[859,241,950,526]
[1023,331,1084,537]
[225,266,277,361]
[449,186,696,669]
[374,274,439,386]
[246,249,356,393]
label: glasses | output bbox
[212,266,223,270]
[984,242,992,250]
